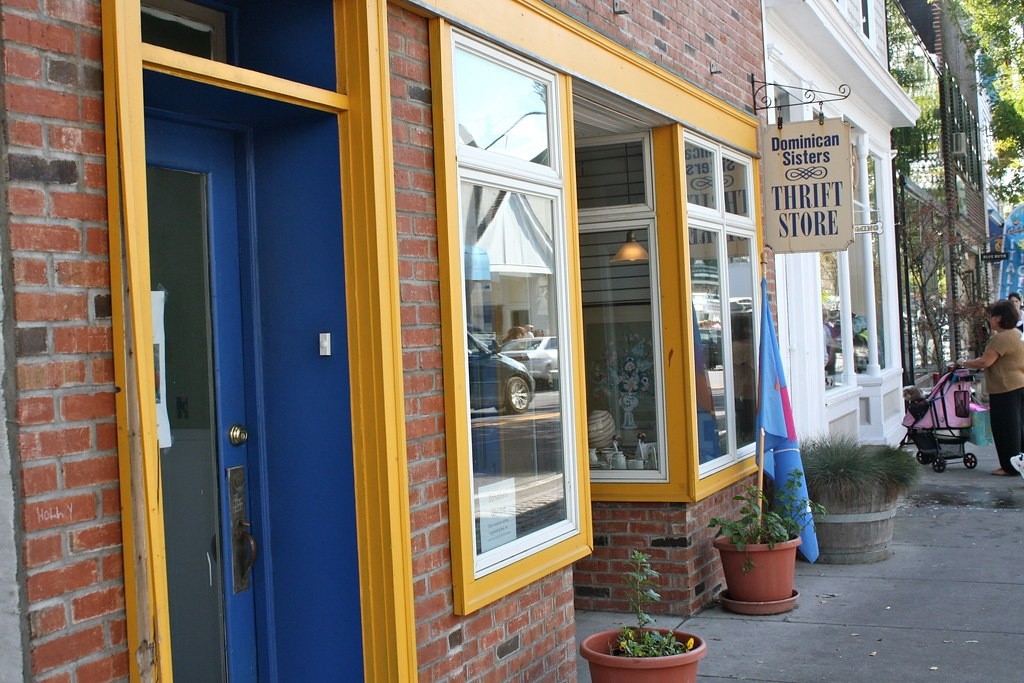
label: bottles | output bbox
[589,447,598,465]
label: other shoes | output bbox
[992,467,1010,475]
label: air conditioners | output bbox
[951,132,968,157]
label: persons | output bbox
[502,324,546,346]
[956,300,1024,477]
[1007,292,1024,341]
[822,307,885,387]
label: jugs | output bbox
[608,453,626,470]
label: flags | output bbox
[754,278,820,565]
[692,300,721,465]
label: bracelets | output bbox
[962,360,966,368]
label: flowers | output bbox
[609,549,695,658]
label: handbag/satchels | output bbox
[959,395,993,447]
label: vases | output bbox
[579,628,708,683]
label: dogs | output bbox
[902,385,931,403]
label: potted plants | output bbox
[799,430,923,565]
[707,466,829,602]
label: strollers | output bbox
[902,367,979,473]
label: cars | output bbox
[499,334,558,390]
[699,326,722,365]
[467,332,537,414]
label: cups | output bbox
[626,459,644,470]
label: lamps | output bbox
[610,143,649,262]
